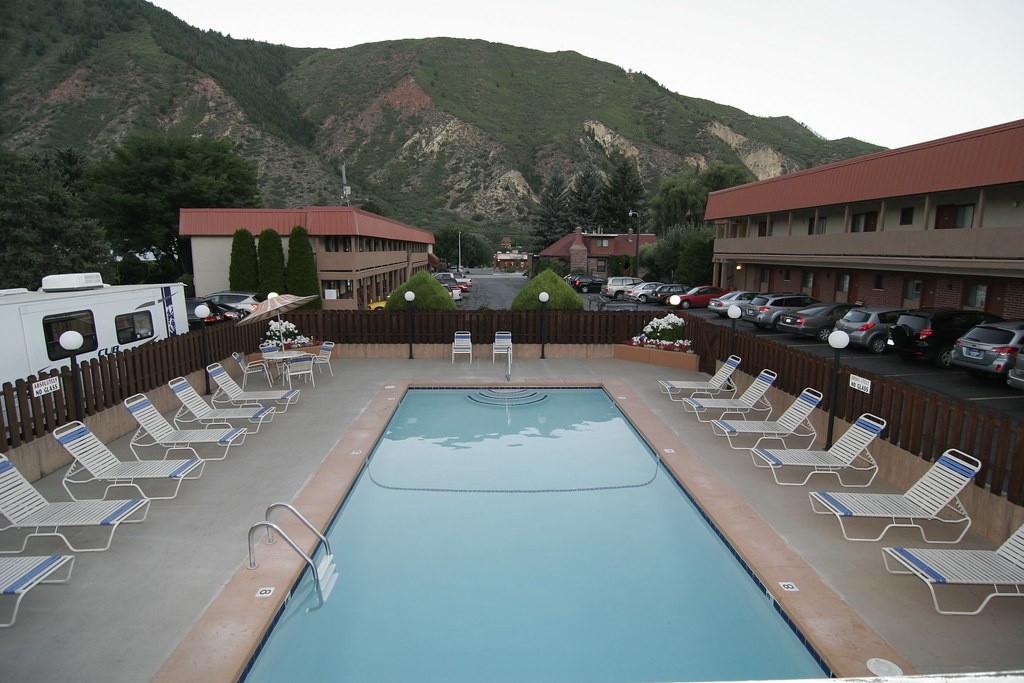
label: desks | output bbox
[262,351,306,385]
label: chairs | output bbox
[124,393,248,461]
[259,343,281,379]
[881,524,1024,615]
[168,377,276,434]
[749,413,886,488]
[0,453,150,554]
[232,352,274,391]
[0,555,75,628]
[682,369,777,423]
[283,353,315,390]
[493,332,512,364]
[710,388,823,451]
[206,363,300,413]
[452,331,472,364]
[658,355,741,401]
[310,341,335,381]
[53,420,206,501]
[807,449,981,544]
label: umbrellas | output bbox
[234,292,318,352]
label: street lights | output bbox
[822,331,850,452]
[629,210,639,277]
[405,291,416,359]
[194,305,211,395]
[726,305,741,390]
[539,292,549,359]
[459,232,461,270]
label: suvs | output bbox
[600,277,645,301]
[885,306,1004,372]
[742,292,821,334]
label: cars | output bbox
[777,302,863,344]
[653,284,693,306]
[708,291,766,318]
[368,301,386,311]
[677,286,729,309]
[432,266,472,301]
[185,292,262,332]
[563,273,607,293]
[951,320,1024,390]
[831,307,917,354]
[627,282,665,304]
